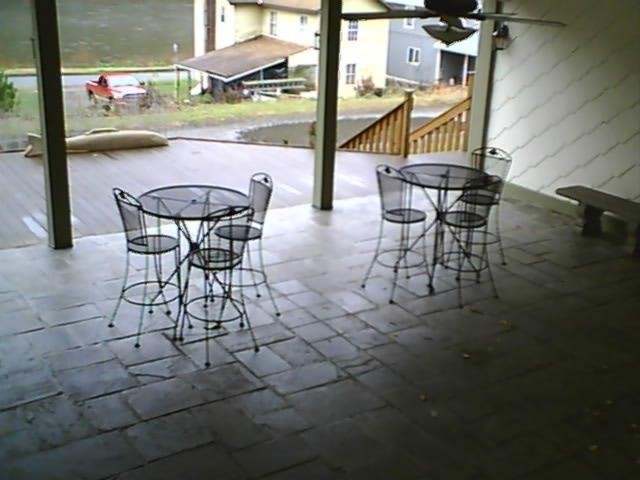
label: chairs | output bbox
[361,164,433,305]
[173,205,260,367]
[446,147,513,284]
[108,187,193,348]
[430,176,503,310]
[203,173,281,329]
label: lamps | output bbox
[492,25,509,50]
[422,17,477,45]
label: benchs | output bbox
[556,185,640,257]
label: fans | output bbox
[339,0,567,28]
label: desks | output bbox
[395,163,501,296]
[134,185,252,342]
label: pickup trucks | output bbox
[85,72,149,106]
